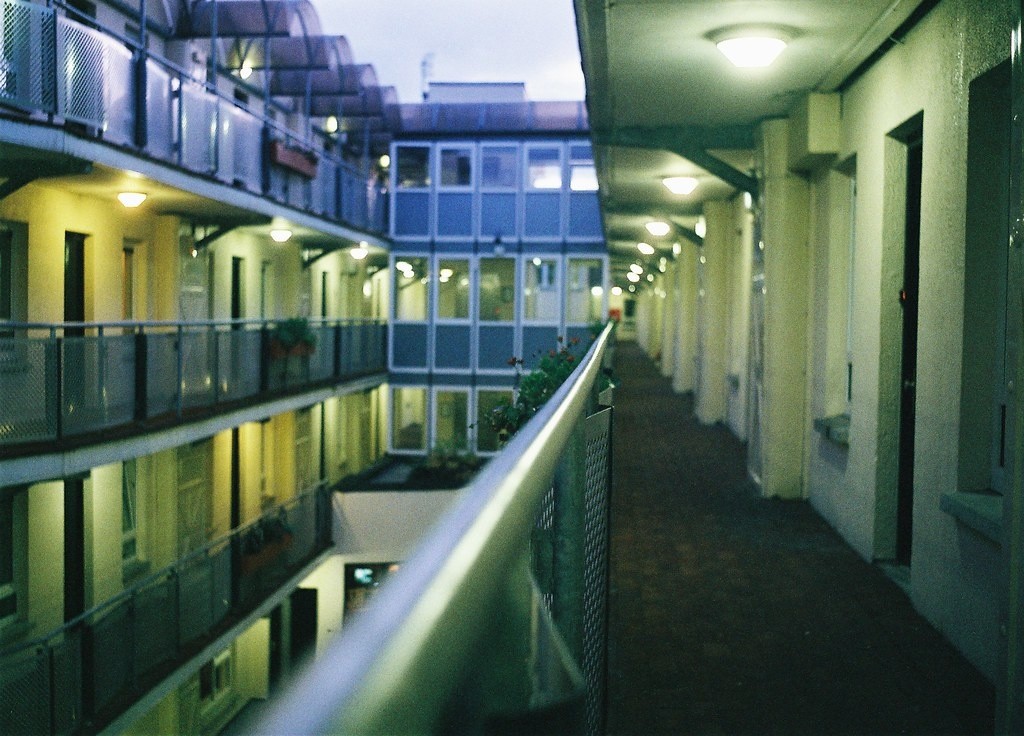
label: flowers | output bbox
[466,335,582,434]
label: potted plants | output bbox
[273,137,319,178]
[265,316,318,358]
[238,509,294,567]
